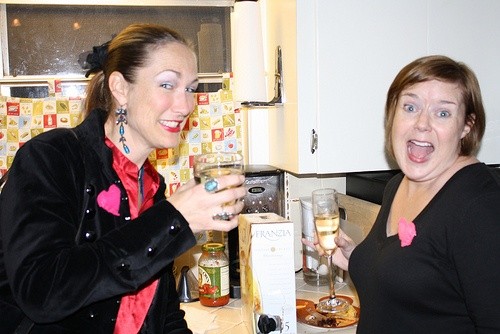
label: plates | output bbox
[296,300,360,332]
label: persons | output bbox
[301,55,500,334]
[0,24,246,334]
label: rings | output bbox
[204,179,218,191]
[216,208,233,221]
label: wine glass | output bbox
[312,188,349,314]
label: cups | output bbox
[193,152,244,221]
[300,198,337,286]
